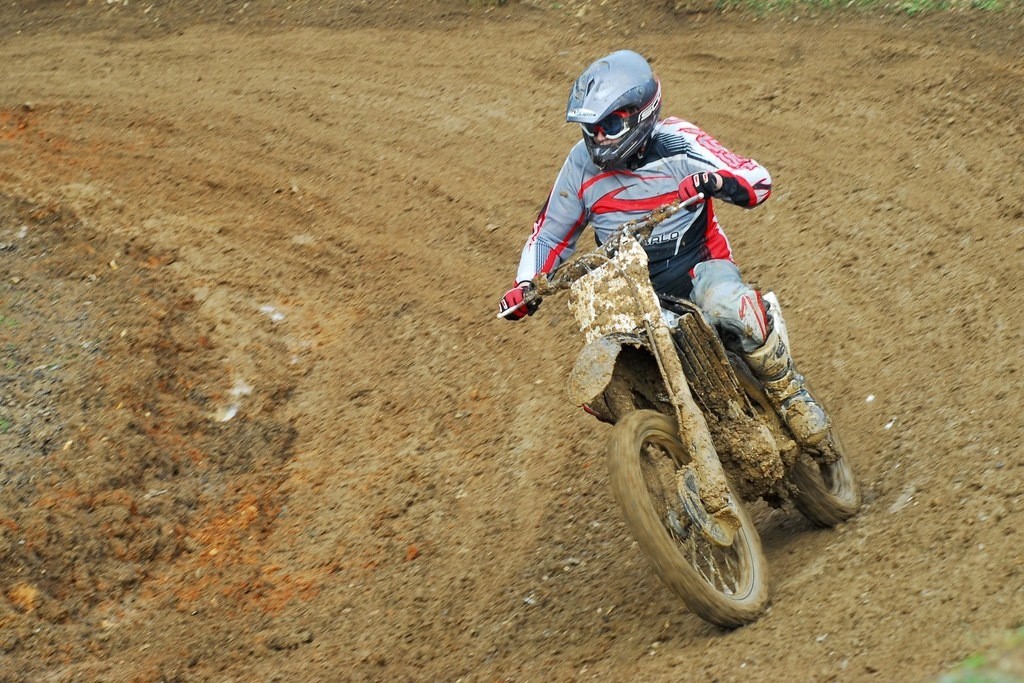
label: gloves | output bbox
[500,279,542,321]
[676,169,725,211]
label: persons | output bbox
[498,49,831,450]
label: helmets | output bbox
[565,49,662,173]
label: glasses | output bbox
[579,113,629,139]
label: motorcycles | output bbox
[497,191,862,629]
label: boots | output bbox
[743,328,831,447]
